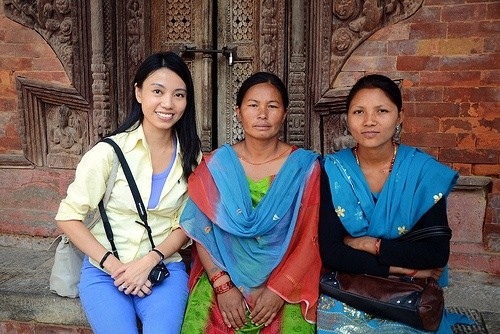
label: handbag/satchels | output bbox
[319,267,445,333]
[47,233,85,298]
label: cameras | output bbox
[148,261,170,285]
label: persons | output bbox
[316,73,460,334]
[180,72,322,334]
[54,51,203,334]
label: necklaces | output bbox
[238,145,293,165]
[354,142,398,172]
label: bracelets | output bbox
[376,238,380,255]
[100,252,112,269]
[214,281,234,295]
[153,249,164,259]
[210,271,229,286]
[408,270,417,277]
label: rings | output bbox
[122,285,127,291]
